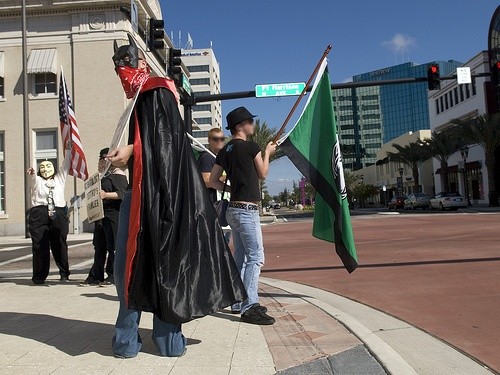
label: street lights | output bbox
[360,174,364,190]
[358,183,361,189]
[397,167,404,194]
[460,146,473,205]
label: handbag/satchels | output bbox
[215,198,228,227]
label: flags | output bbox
[58,70,87,181]
[276,56,358,274]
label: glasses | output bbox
[212,137,225,142]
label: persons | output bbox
[26,138,75,285]
[107,33,247,358]
[78,107,277,324]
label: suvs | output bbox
[387,196,409,210]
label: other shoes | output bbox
[79,277,114,286]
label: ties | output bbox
[48,187,57,221]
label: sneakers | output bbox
[232,304,275,325]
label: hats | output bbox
[225,106,257,130]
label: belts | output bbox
[227,202,257,210]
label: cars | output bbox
[267,203,270,209]
[274,205,280,209]
[429,191,464,211]
[403,193,431,210]
[266,204,269,209]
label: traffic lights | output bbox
[426,63,441,90]
[150,17,164,51]
[170,47,181,77]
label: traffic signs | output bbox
[255,83,306,98]
